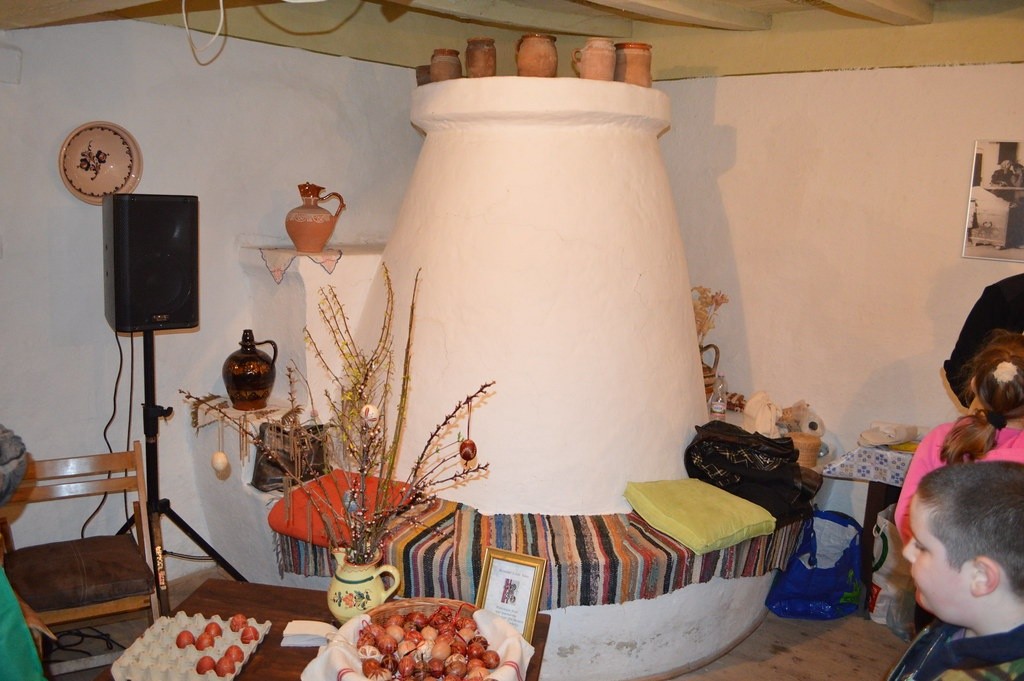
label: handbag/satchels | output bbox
[766,507,865,622]
[867,503,917,637]
[251,423,334,491]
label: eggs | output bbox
[356,606,500,681]
[361,404,379,428]
[461,440,477,461]
[176,614,260,676]
[212,451,229,470]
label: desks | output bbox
[92,578,550,681]
[823,445,914,609]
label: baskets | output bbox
[364,599,476,622]
[787,432,823,469]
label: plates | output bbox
[59,121,143,206]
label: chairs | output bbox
[0,440,161,676]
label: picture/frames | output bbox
[961,139,1024,264]
[475,546,546,645]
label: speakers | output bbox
[113,194,199,332]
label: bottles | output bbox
[708,372,727,423]
[222,329,278,411]
[285,182,346,253]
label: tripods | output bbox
[113,331,250,613]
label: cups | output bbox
[431,49,462,82]
[614,43,652,88]
[416,65,430,86]
[516,34,558,77]
[572,37,616,81]
[466,37,497,78]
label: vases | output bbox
[327,543,401,626]
[698,343,720,403]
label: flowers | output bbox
[691,284,730,345]
[179,260,498,565]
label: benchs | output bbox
[242,478,803,681]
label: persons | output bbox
[885,460,1024,681]
[894,333,1024,542]
[943,273,1024,407]
